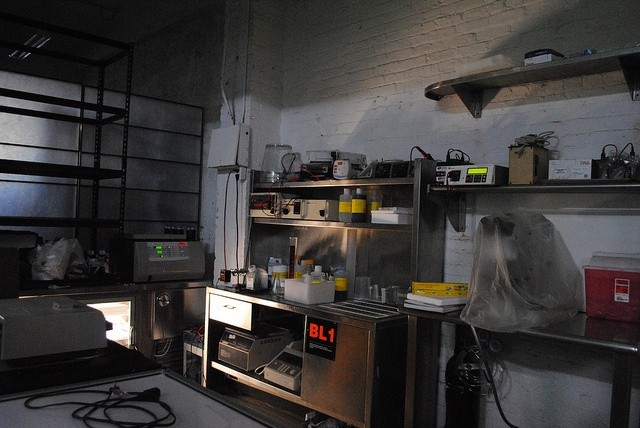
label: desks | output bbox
[397,293,639,427]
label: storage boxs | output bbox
[582,252,640,323]
[285,277,336,304]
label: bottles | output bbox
[334,269,348,302]
[268,257,282,287]
[311,266,324,280]
[246,265,261,290]
[352,188,368,223]
[367,187,383,224]
[338,188,351,222]
[293,256,302,280]
[272,265,290,292]
[303,260,315,280]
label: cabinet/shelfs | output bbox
[1,12,134,298]
[199,158,448,427]
[424,50,640,231]
